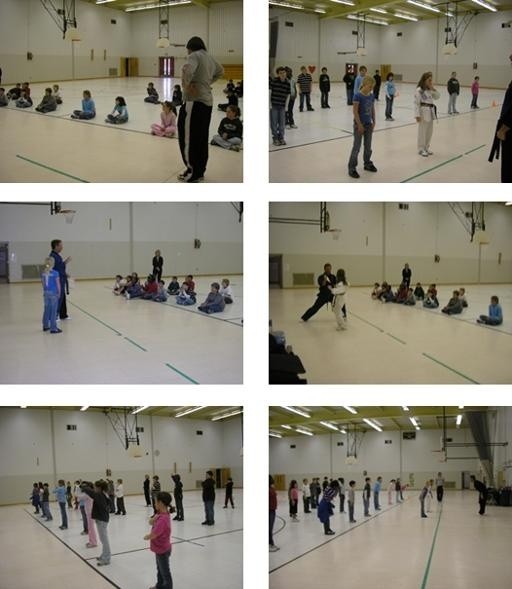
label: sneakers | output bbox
[418,149,432,156]
[349,169,360,178]
[286,124,298,129]
[273,139,286,146]
[363,165,377,173]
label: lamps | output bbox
[94,0,196,15]
[267,405,470,442]
[18,406,243,426]
[269,0,501,30]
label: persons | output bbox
[270,470,434,552]
[112,250,233,315]
[470,475,488,515]
[269,319,308,383]
[298,263,347,323]
[0,36,242,182]
[327,269,351,331]
[48,239,72,321]
[435,472,444,505]
[477,295,503,325]
[39,257,63,333]
[269,53,512,183]
[28,470,236,589]
[371,262,468,315]
